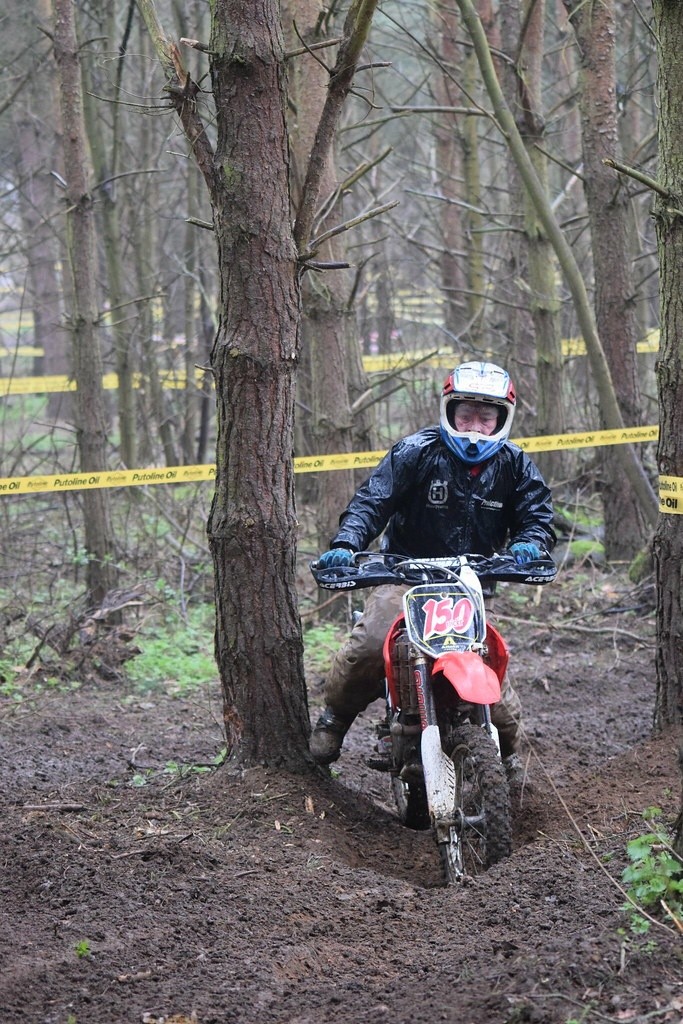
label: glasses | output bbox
[454,403,500,420]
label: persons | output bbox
[310,360,557,770]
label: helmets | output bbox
[440,361,517,467]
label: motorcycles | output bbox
[310,552,558,887]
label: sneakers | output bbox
[501,751,530,787]
[309,704,359,765]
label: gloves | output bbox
[319,547,359,578]
[507,542,539,571]
